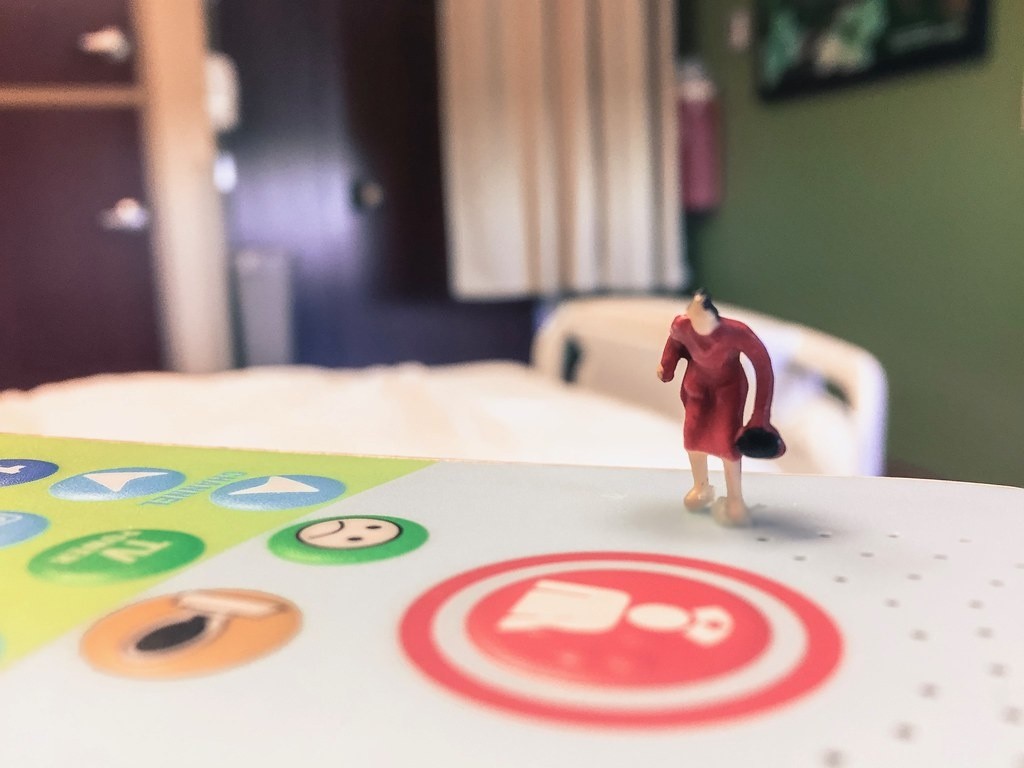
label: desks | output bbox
[0,358,1024,768]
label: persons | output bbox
[656,287,787,528]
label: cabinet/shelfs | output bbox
[0,0,224,390]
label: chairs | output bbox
[535,300,888,484]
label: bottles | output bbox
[679,59,725,208]
